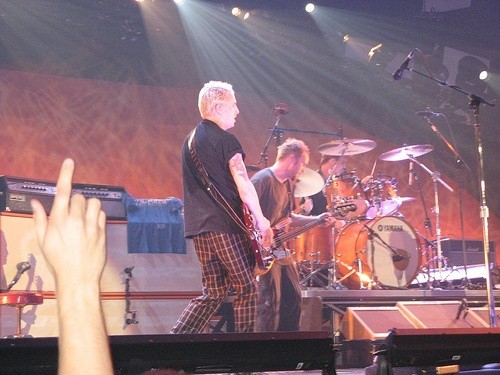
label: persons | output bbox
[29,157,114,375]
[299,152,346,326]
[168,80,274,334]
[248,136,338,332]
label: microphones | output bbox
[392,254,403,262]
[120,267,133,277]
[16,262,31,271]
[415,110,442,117]
[391,50,415,81]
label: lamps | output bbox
[366,42,397,68]
[302,2,317,15]
[459,54,489,80]
[419,57,450,82]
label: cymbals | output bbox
[316,138,376,156]
[379,144,434,161]
[289,167,325,198]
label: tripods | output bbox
[406,155,465,290]
[298,149,382,291]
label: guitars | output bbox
[255,204,357,275]
[242,202,266,270]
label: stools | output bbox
[0,290,43,339]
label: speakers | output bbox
[340,301,499,339]
[432,238,494,269]
[0,212,210,334]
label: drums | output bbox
[363,174,403,219]
[334,216,423,290]
[284,226,333,269]
[323,175,369,219]
